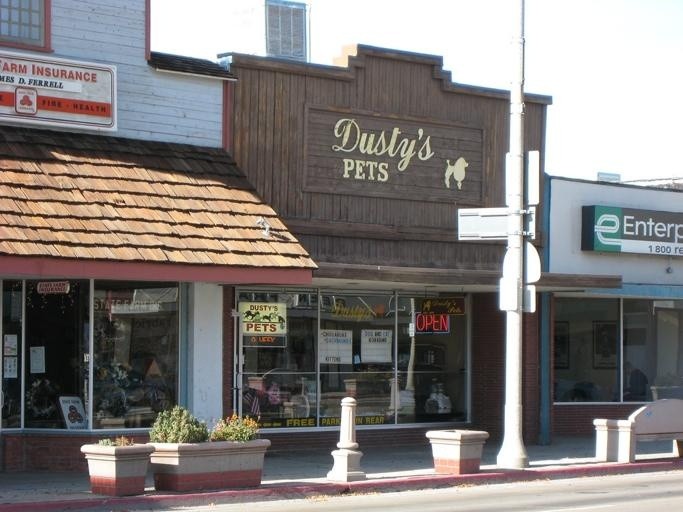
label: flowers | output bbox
[149,405,259,443]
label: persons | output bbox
[613,361,648,402]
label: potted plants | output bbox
[78,436,156,497]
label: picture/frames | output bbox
[554,321,570,369]
[592,320,620,370]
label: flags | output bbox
[242,389,260,419]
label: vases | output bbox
[423,430,489,476]
[146,439,271,491]
